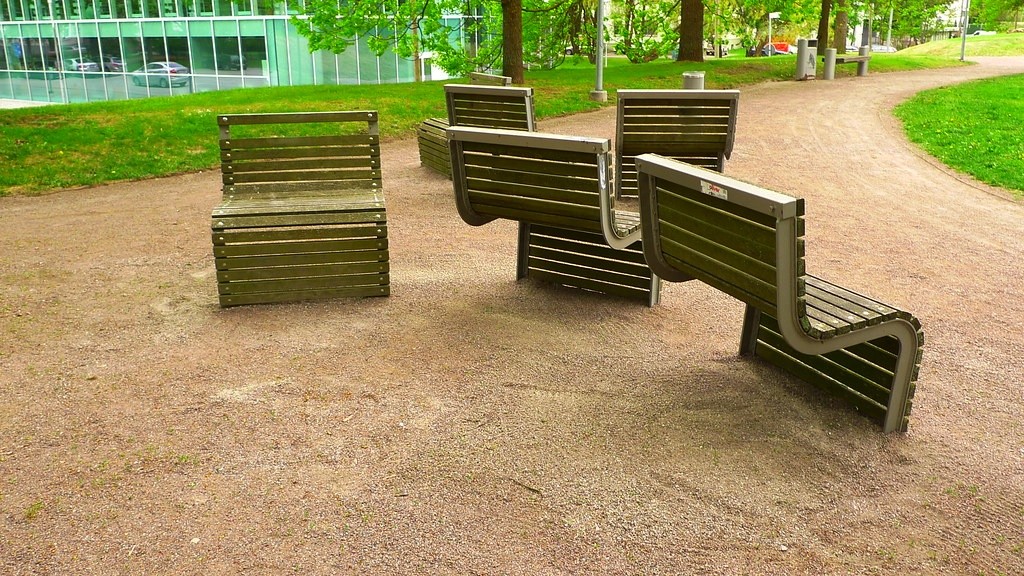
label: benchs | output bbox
[633,152,924,437]
[443,83,536,133]
[447,125,662,309]
[211,111,391,308]
[822,56,872,64]
[416,72,512,181]
[614,87,740,201]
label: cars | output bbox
[563,42,595,56]
[130,61,189,88]
[218,53,247,71]
[749,41,795,57]
[21,45,126,72]
